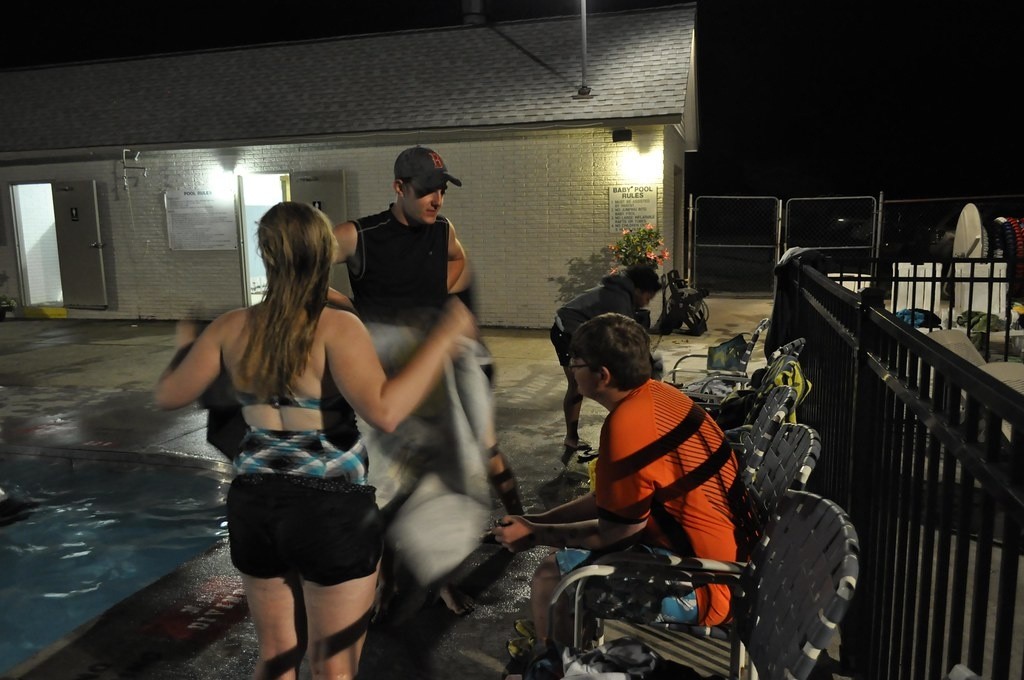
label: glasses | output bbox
[568,357,601,372]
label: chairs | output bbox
[534,318,863,680]
[978,362,1023,444]
[925,327,986,367]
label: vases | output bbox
[634,308,650,330]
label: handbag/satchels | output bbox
[707,333,752,385]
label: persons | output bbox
[550,265,664,450]
[494,314,740,680]
[327,143,523,517]
[152,202,470,680]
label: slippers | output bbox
[578,448,599,461]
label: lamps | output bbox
[612,127,632,143]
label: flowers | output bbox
[606,223,670,277]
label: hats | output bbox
[393,147,462,197]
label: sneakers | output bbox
[514,619,536,638]
[506,638,536,658]
